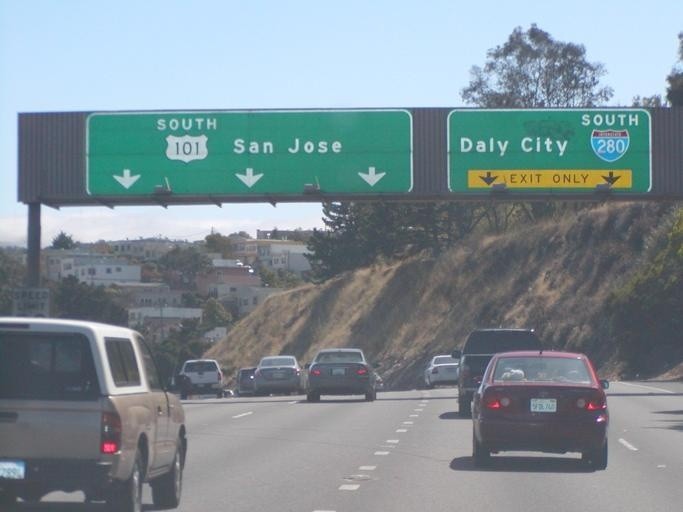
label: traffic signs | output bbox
[84,109,413,194]
[447,110,653,192]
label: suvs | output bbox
[452,328,544,414]
[178,358,222,398]
[0,316,188,512]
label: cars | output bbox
[424,354,459,386]
[236,354,301,394]
[302,348,382,401]
[471,350,609,469]
